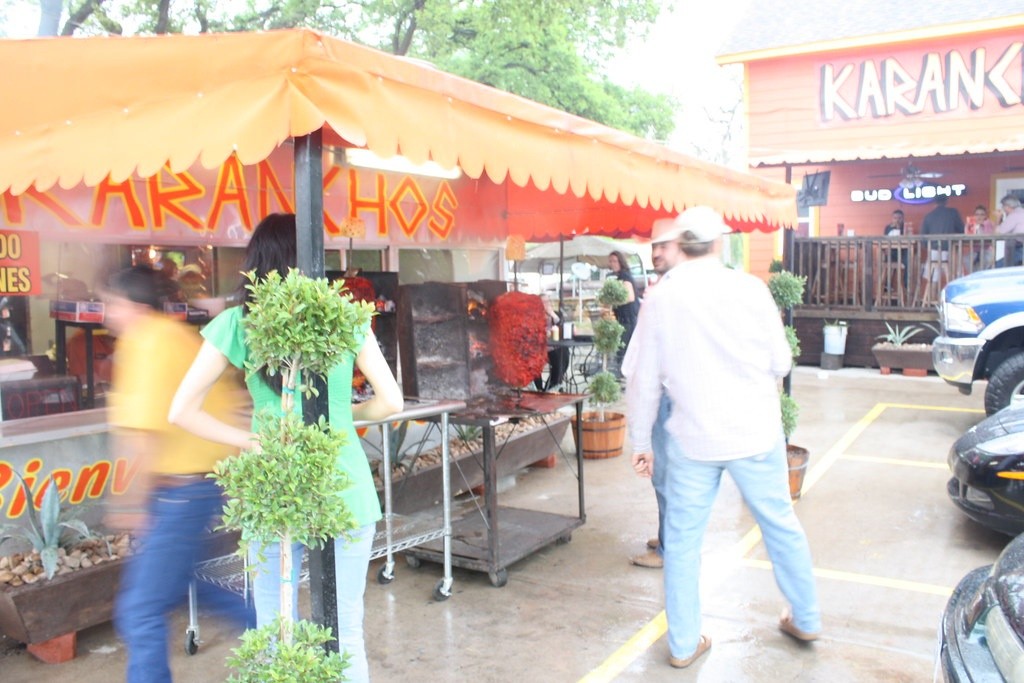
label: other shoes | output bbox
[648,538,660,549]
[634,553,662,567]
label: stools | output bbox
[810,258,955,310]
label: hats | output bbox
[630,220,677,244]
[674,207,731,244]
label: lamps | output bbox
[330,146,460,179]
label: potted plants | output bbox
[821,317,849,355]
[569,276,629,460]
[765,270,811,499]
[870,320,939,371]
[0,470,242,645]
[358,411,571,513]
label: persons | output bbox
[626,206,820,669]
[167,213,403,683]
[626,221,688,567]
[879,194,1024,307]
[608,250,640,381]
[93,266,263,683]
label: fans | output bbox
[867,156,943,181]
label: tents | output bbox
[0,27,798,543]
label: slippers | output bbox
[670,635,710,669]
[784,615,819,642]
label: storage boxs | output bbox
[161,302,188,322]
[49,299,105,323]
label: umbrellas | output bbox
[523,228,644,325]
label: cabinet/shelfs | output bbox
[184,394,468,655]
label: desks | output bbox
[0,351,83,419]
[54,315,214,409]
[549,339,598,395]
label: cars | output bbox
[931,265,1024,419]
[630,264,660,295]
[947,399,1024,538]
[939,531,1024,683]
